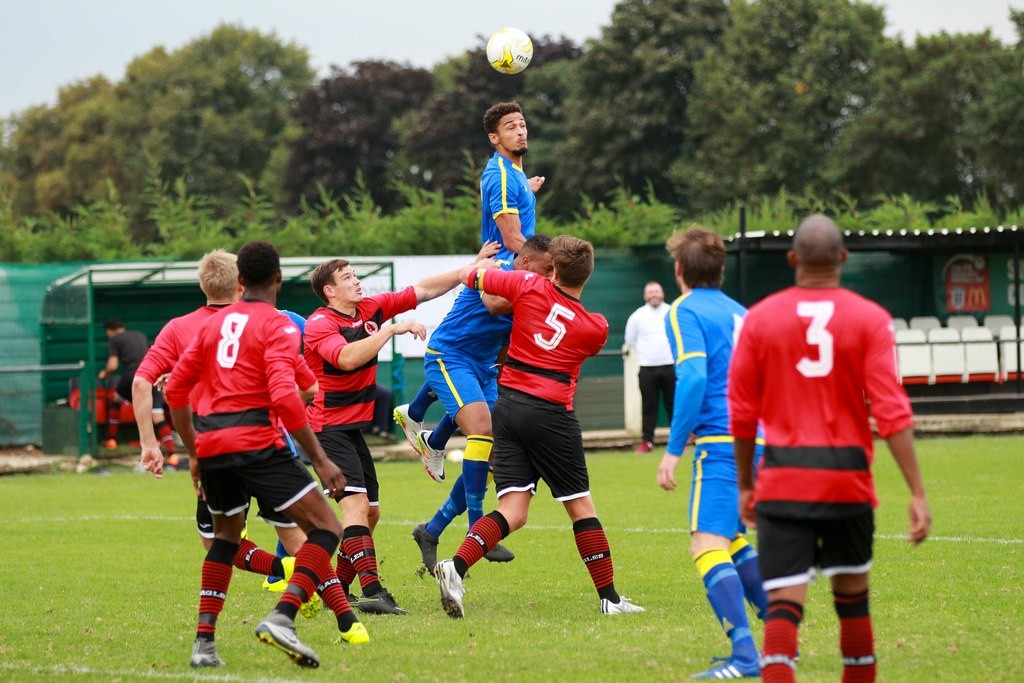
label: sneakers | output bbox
[190,639,224,668]
[254,614,319,668]
[434,560,465,617]
[323,593,360,607]
[692,656,760,679]
[334,621,369,644]
[412,523,438,576]
[262,576,287,592]
[391,404,425,456]
[358,588,408,614]
[281,556,320,618]
[600,595,645,614]
[417,430,446,483]
[463,531,514,563]
[635,440,653,453]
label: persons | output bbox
[131,248,370,644]
[624,281,695,453]
[97,319,179,466]
[656,222,800,679]
[303,239,502,616]
[433,234,646,619]
[162,241,347,668]
[392,103,547,484]
[411,235,555,578]
[726,214,934,683]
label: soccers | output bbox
[486,27,534,75]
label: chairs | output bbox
[893,312,1024,386]
